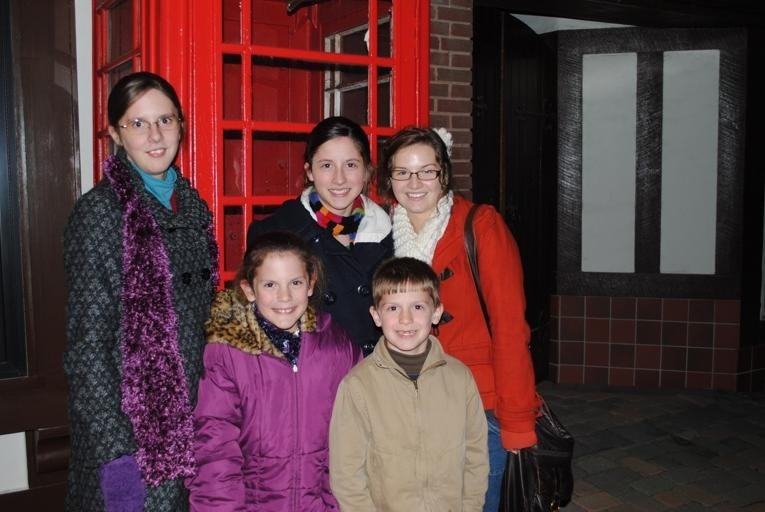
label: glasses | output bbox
[119,114,181,130]
[390,168,443,180]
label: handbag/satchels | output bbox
[498,396,574,510]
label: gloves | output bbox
[101,456,146,512]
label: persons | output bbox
[384,126,542,511]
[328,257,491,511]
[62,71,220,511]
[247,116,394,357]
[185,230,364,511]
